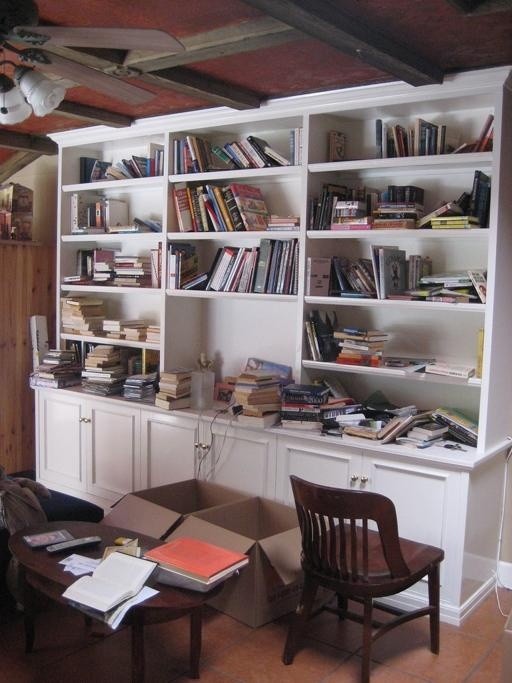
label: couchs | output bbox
[0,462,106,627]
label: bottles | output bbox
[190,352,217,410]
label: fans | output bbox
[0,0,189,135]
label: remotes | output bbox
[46,536,101,553]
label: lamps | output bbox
[0,58,73,129]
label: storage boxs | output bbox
[163,496,334,629]
[98,478,258,541]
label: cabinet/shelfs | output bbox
[55,126,169,382]
[279,429,512,627]
[140,404,278,500]
[169,104,306,383]
[306,85,512,451]
[34,386,140,505]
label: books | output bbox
[2,182,33,240]
[375,116,494,158]
[143,533,250,591]
[310,170,491,230]
[306,245,486,304]
[305,310,484,385]
[330,130,345,161]
[29,342,159,404]
[70,194,162,235]
[80,142,163,183]
[65,584,160,630]
[60,551,159,613]
[60,296,161,343]
[167,238,299,295]
[63,242,162,288]
[280,373,478,449]
[173,127,302,175]
[155,367,194,410]
[213,358,296,429]
[172,182,300,232]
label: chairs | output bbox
[281,473,446,683]
